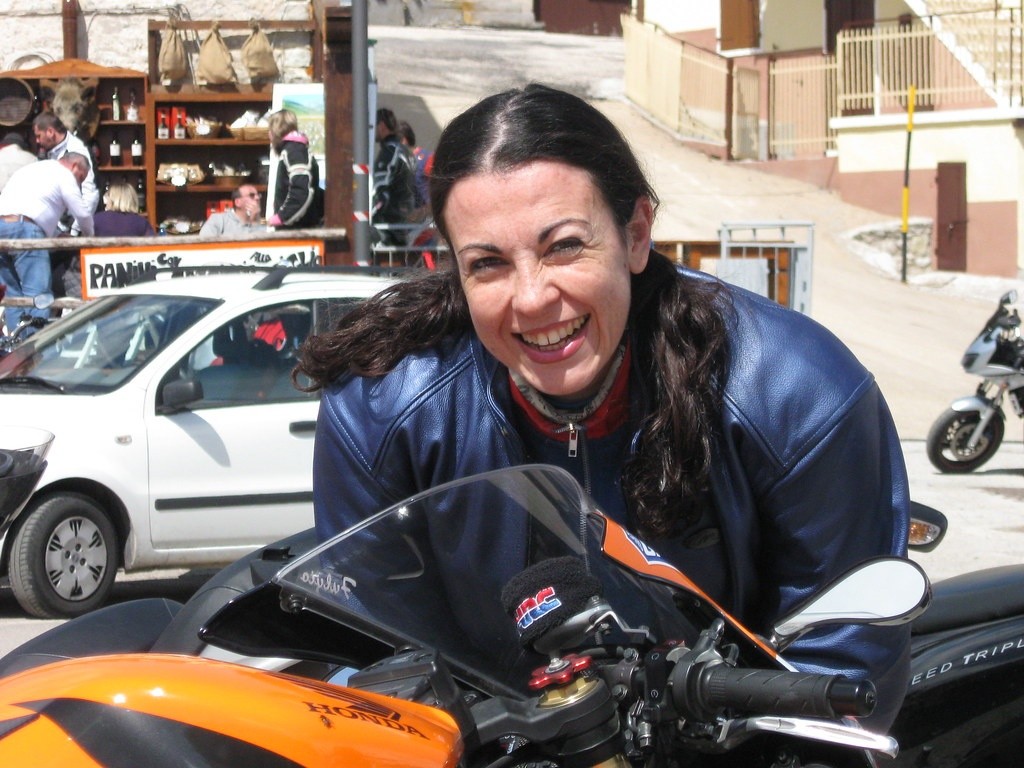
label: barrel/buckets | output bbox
[256,159,269,184]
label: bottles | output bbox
[225,203,230,211]
[210,203,216,215]
[110,131,120,166]
[112,86,122,121]
[131,130,142,166]
[158,109,169,140]
[174,107,185,139]
[126,90,139,121]
[36,144,48,159]
[90,134,102,165]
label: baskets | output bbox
[226,124,269,140]
[186,120,223,139]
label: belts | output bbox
[0,215,34,222]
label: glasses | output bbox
[242,193,262,199]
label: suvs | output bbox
[0,266,411,621]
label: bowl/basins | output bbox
[215,175,248,187]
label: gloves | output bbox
[269,215,283,227]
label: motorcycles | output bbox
[0,465,1024,768]
[926,291,1024,474]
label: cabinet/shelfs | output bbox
[0,59,277,235]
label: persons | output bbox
[198,183,275,235]
[267,109,320,228]
[312,86,910,768]
[33,113,100,298]
[0,153,94,340]
[94,182,155,237]
[373,109,438,265]
[1,133,38,194]
